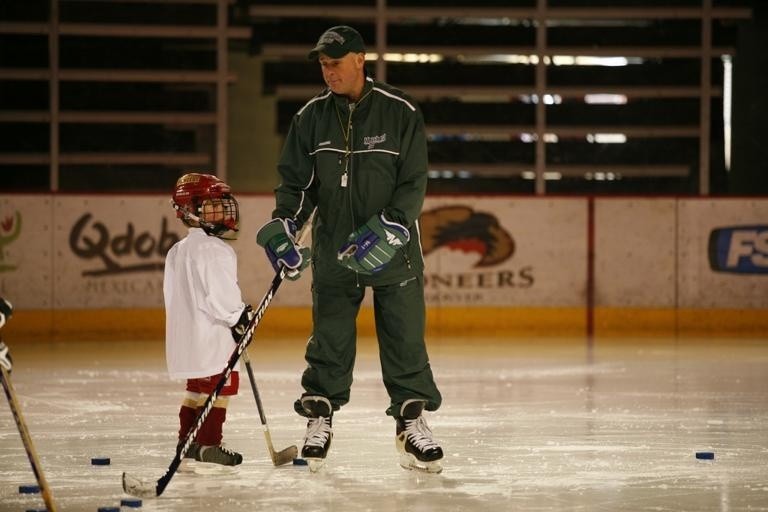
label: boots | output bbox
[176,442,244,466]
[394,402,444,462]
[294,397,335,461]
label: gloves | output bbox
[229,305,256,345]
[336,208,410,278]
[0,296,13,330]
[257,219,312,281]
[0,339,14,375]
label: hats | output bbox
[306,24,367,59]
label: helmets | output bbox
[171,172,233,226]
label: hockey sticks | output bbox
[242,347,298,466]
[122,208,316,499]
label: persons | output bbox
[256,25,444,461]
[162,171,258,466]
